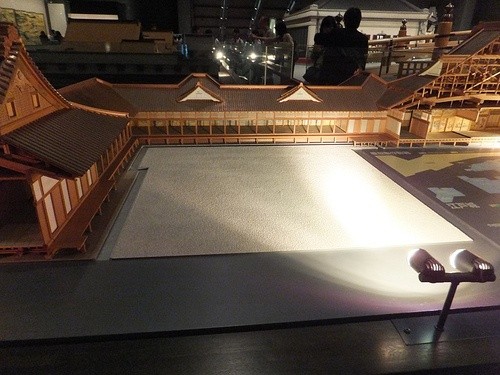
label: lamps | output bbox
[390,249,497,345]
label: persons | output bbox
[39,31,49,46]
[249,21,296,78]
[302,6,369,86]
[310,15,341,68]
[256,14,276,45]
[243,28,261,50]
[214,35,220,46]
[232,30,243,53]
[50,29,64,44]
